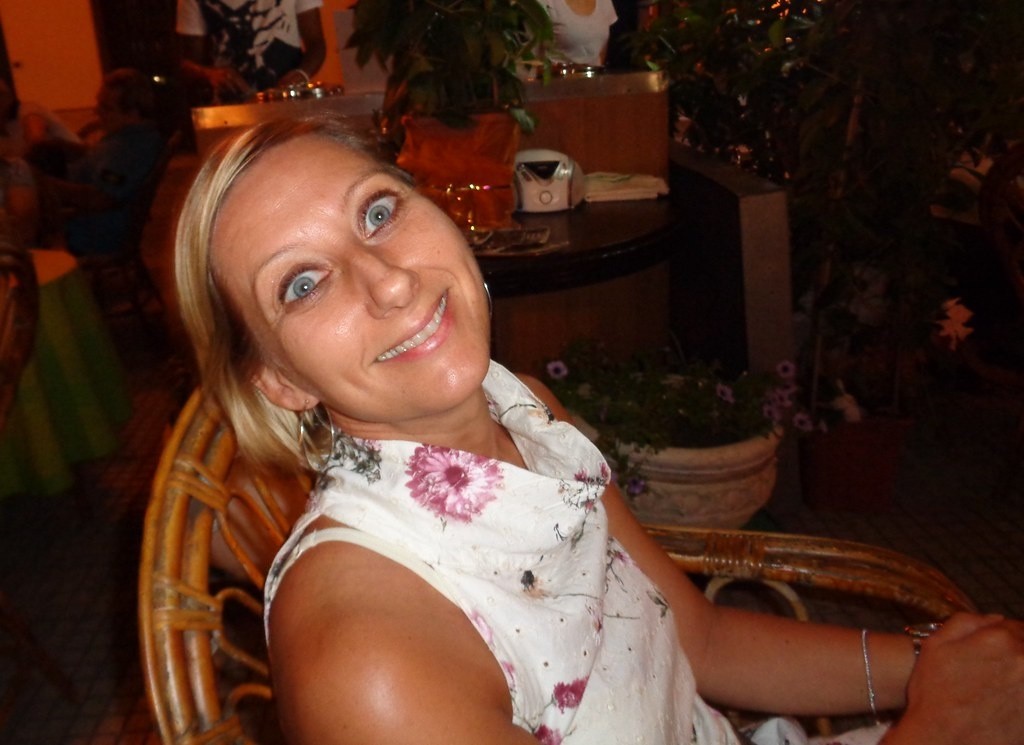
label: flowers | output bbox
[546,343,816,499]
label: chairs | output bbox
[143,367,974,745]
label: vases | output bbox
[572,376,782,529]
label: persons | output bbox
[534,0,619,75]
[0,0,327,373]
[158,114,1024,745]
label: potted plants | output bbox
[349,0,553,230]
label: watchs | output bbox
[904,619,942,660]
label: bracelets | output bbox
[861,629,875,715]
[292,68,310,85]
